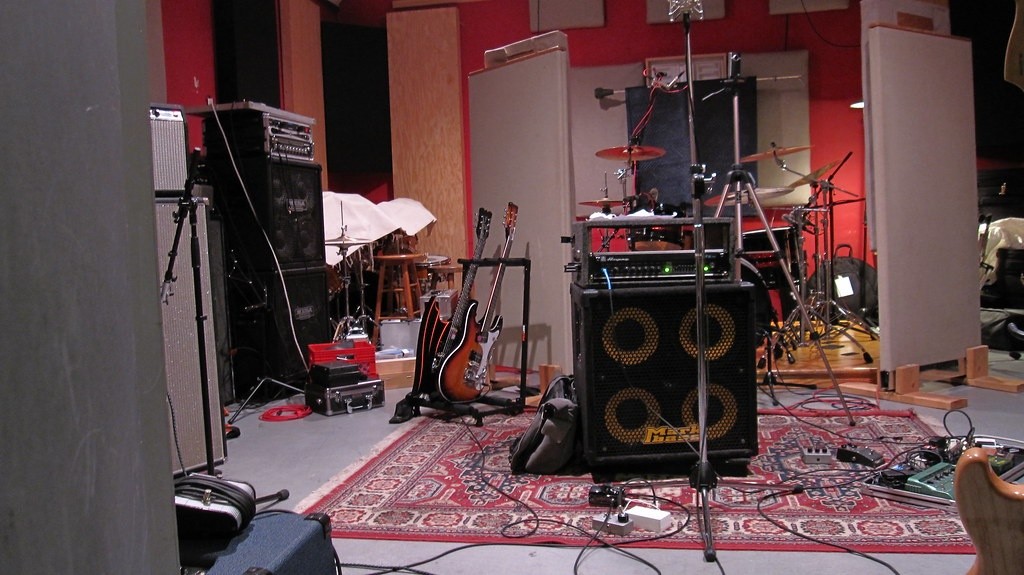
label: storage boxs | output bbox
[306,378,386,417]
[307,341,377,376]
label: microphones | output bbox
[782,214,815,235]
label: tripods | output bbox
[329,242,382,343]
[227,250,310,425]
[605,9,881,562]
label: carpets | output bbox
[289,409,973,553]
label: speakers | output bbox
[570,278,759,468]
[155,195,227,477]
[202,155,326,273]
[224,264,332,403]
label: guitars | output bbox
[409,207,494,402]
[437,202,519,404]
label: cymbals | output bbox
[703,187,795,208]
[739,145,814,163]
[595,145,667,162]
[578,198,624,208]
[788,159,841,189]
[325,236,373,249]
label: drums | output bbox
[741,225,802,288]
[414,254,452,295]
[627,202,685,251]
[740,258,773,350]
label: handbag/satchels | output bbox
[509,377,579,474]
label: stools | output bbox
[371,253,426,342]
